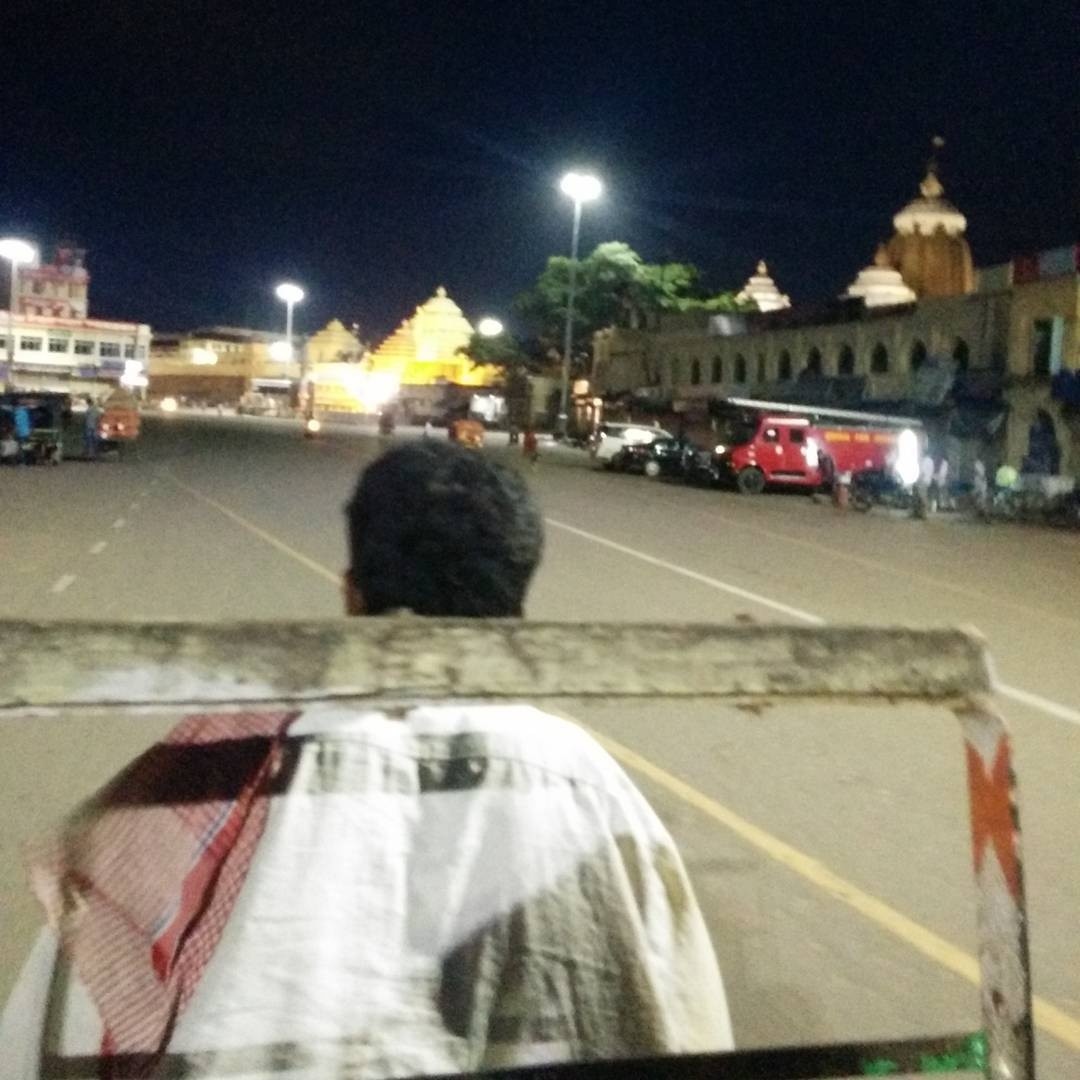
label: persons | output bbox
[423,414,433,441]
[9,399,35,467]
[304,410,314,440]
[522,425,536,457]
[80,398,101,461]
[833,449,996,525]
[510,421,519,443]
[645,413,660,429]
[177,392,244,418]
[815,449,835,496]
[620,407,632,423]
[0,441,736,1076]
[555,406,568,441]
[377,407,394,448]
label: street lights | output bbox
[0,239,35,315]
[276,280,307,361]
[553,169,610,441]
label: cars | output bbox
[4,373,924,501]
